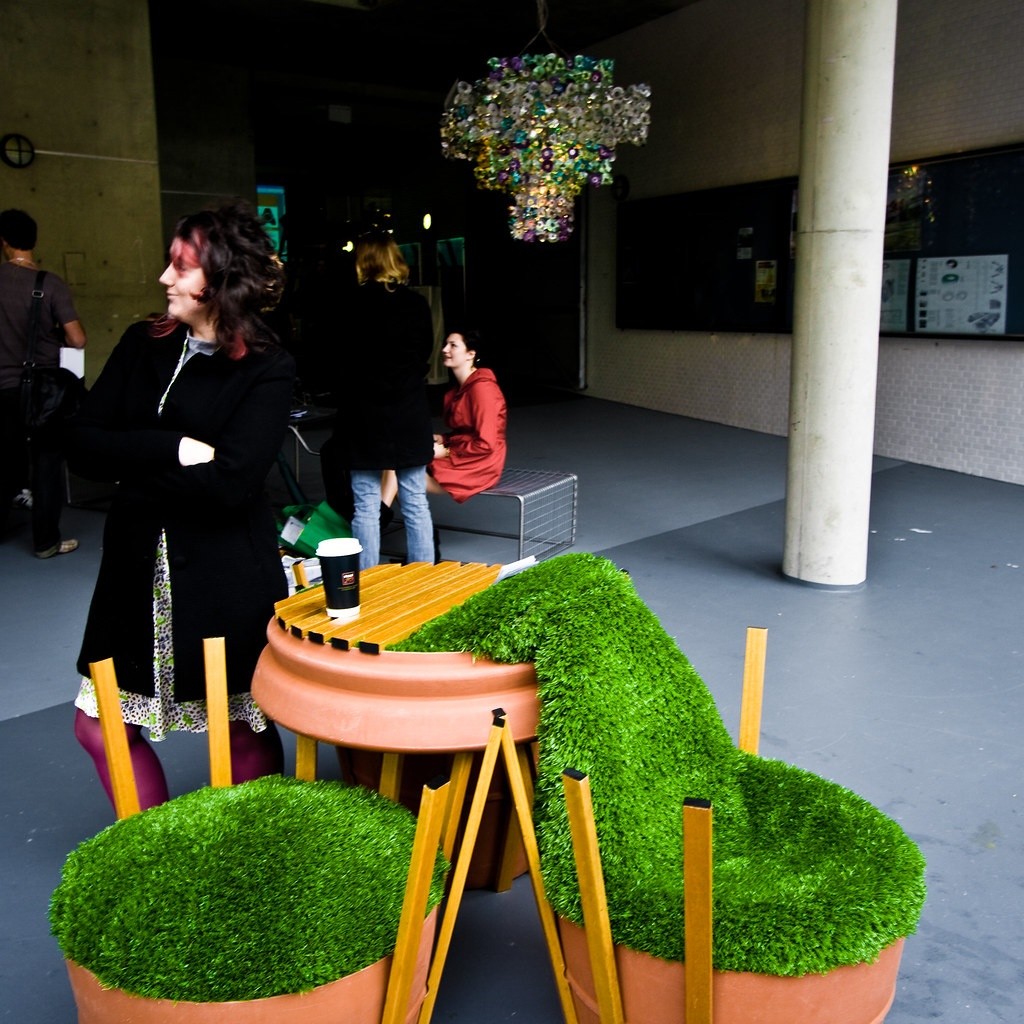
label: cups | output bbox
[315,537,364,609]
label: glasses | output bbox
[187,206,233,277]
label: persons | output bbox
[75,205,294,823]
[322,231,434,572]
[380,324,506,567]
[0,207,85,559]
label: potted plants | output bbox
[47,773,449,1024]
[251,549,629,894]
[528,748,926,1024]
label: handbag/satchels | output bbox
[276,498,351,559]
[16,365,89,440]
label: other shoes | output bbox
[35,539,78,558]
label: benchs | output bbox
[480,467,578,562]
